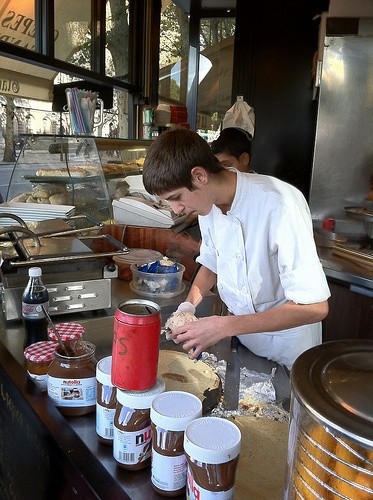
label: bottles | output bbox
[22,267,50,351]
[24,318,242,500]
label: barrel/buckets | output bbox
[285,341,373,500]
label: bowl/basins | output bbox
[112,246,163,281]
[129,260,185,294]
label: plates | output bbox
[129,280,185,299]
[0,200,77,226]
[344,206,373,222]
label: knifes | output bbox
[222,335,240,411]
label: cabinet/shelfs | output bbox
[320,275,373,344]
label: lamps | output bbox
[325,16,359,37]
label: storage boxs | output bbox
[151,126,172,141]
[170,106,188,123]
[176,123,191,130]
[321,219,336,232]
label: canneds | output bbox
[142,107,156,140]
[24,322,101,416]
[95,299,241,500]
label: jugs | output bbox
[65,88,104,135]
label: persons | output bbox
[213,127,259,174]
[144,128,331,413]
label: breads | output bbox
[7,180,130,211]
[36,157,145,180]
